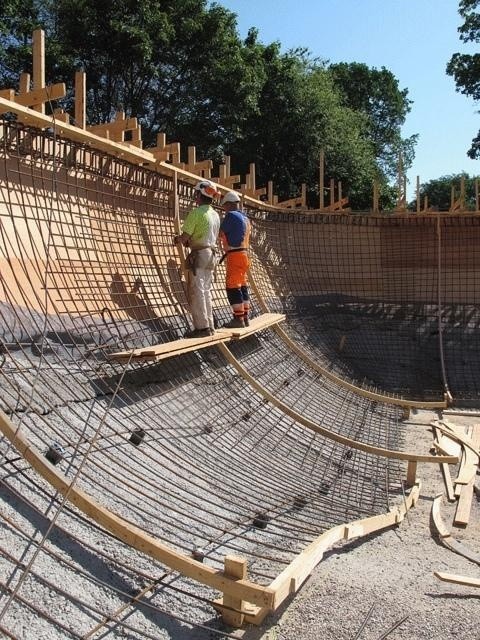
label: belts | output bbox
[219,248,247,264]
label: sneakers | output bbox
[211,327,215,335]
[223,319,245,328]
[187,327,212,338]
[244,319,250,326]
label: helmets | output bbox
[192,181,217,199]
[221,191,241,204]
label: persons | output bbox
[173,180,221,338]
[216,192,251,329]
